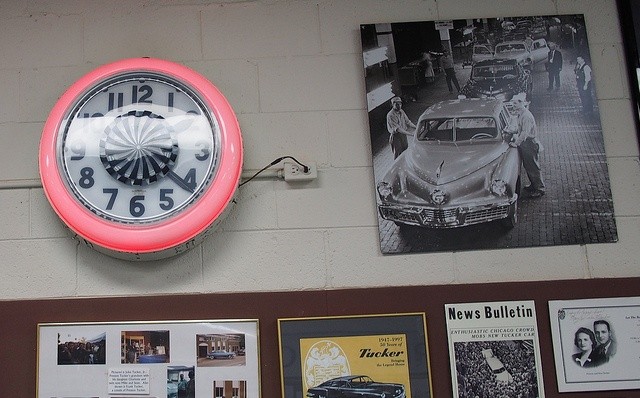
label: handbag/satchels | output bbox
[544,60,552,72]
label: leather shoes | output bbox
[524,184,534,190]
[531,190,545,198]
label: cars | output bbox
[207,350,236,360]
[471,39,550,68]
[481,348,513,385]
[456,59,533,108]
[504,19,549,39]
[377,99,522,230]
[305,375,407,398]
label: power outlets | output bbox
[284,162,318,182]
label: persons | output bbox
[569,327,605,371]
[419,50,436,85]
[440,49,461,94]
[574,56,596,114]
[544,42,563,91]
[595,318,617,362]
[58,338,106,364]
[125,341,159,363]
[502,93,549,198]
[386,95,416,161]
[186,370,195,398]
[576,19,588,55]
[177,373,187,398]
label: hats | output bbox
[392,97,401,103]
[510,95,525,102]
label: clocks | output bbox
[38,58,245,264]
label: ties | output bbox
[600,344,606,358]
[550,51,553,59]
